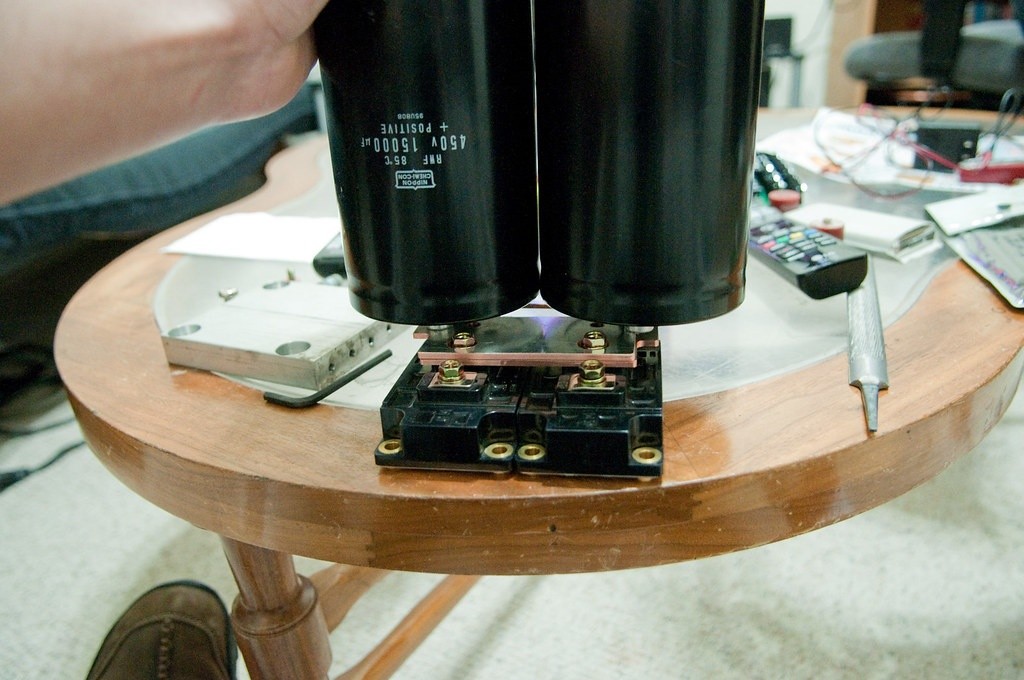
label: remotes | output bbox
[747,218,868,299]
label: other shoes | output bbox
[86,580,237,680]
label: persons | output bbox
[1,0,338,680]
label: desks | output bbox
[53,106,1023,680]
